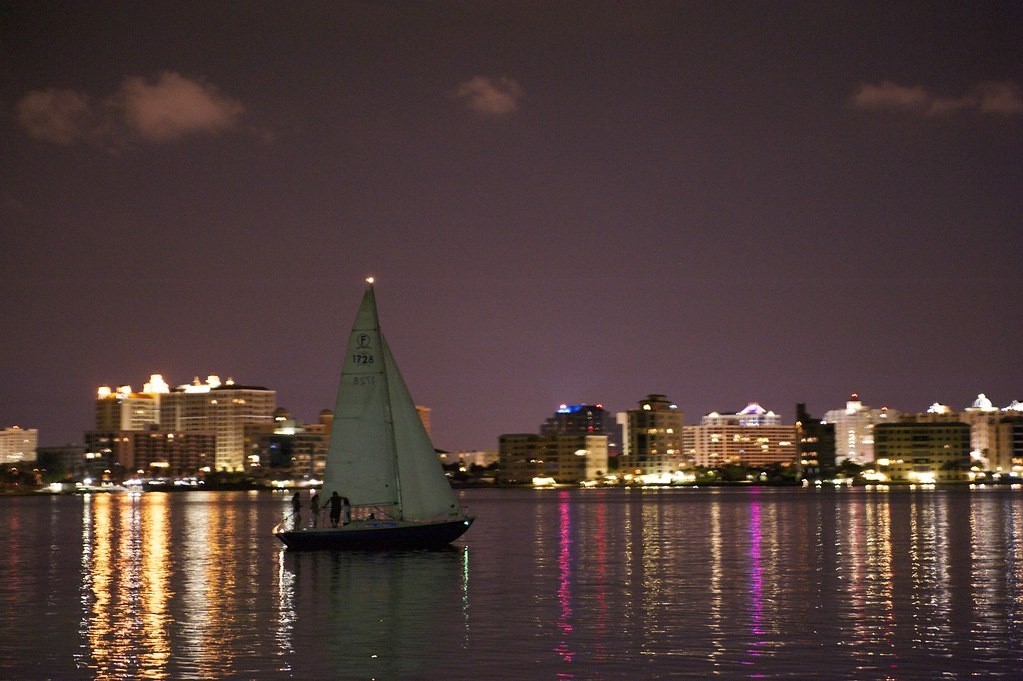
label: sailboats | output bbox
[272,278,477,551]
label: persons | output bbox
[367,512,375,519]
[292,492,303,530]
[343,497,352,525]
[323,490,346,527]
[309,495,320,529]
